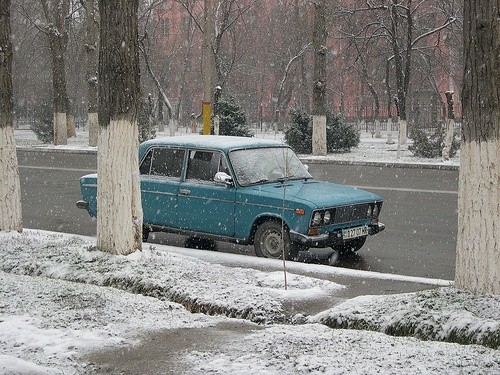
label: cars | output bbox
[75,135,387,260]
